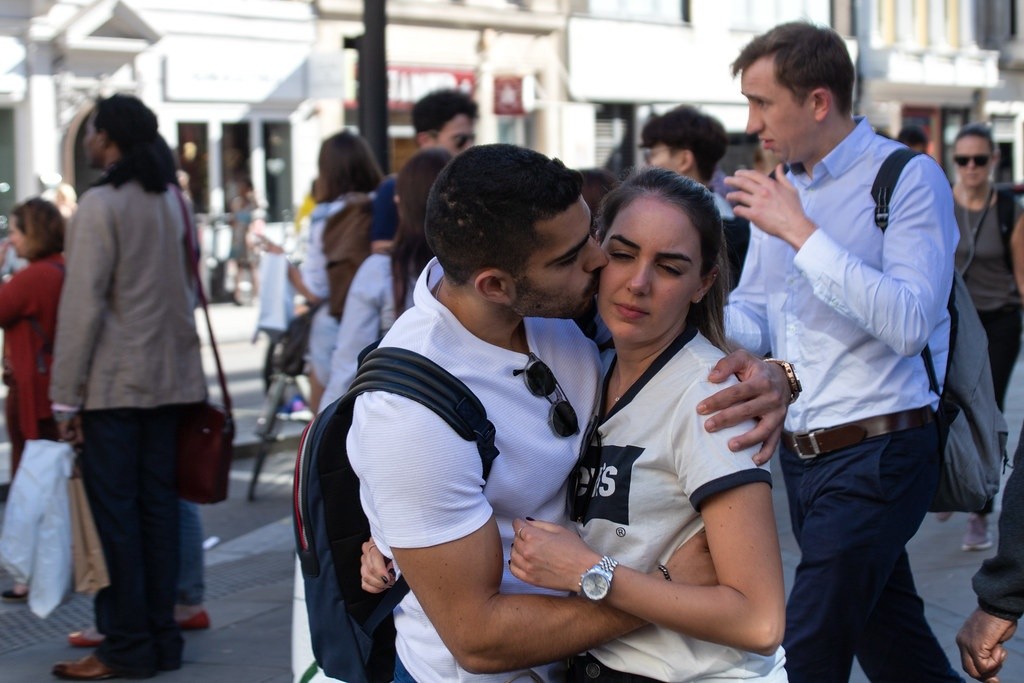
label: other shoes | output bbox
[0,587,28,601]
[275,397,314,421]
[176,611,208,629]
[68,631,107,647]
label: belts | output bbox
[780,406,933,461]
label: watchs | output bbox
[763,356,803,404]
[577,555,618,600]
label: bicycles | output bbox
[245,326,312,504]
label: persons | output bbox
[267,19,1024,683]
[227,175,268,309]
[0,91,210,683]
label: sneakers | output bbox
[963,516,992,549]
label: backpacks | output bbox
[293,333,501,683]
[871,143,1004,517]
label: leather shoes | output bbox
[52,656,133,680]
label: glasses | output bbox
[952,154,994,167]
[645,147,680,165]
[428,129,474,149]
[569,417,602,523]
[512,352,581,438]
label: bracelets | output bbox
[658,563,672,581]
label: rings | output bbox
[368,544,376,552]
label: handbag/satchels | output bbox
[0,436,109,620]
[174,396,235,501]
